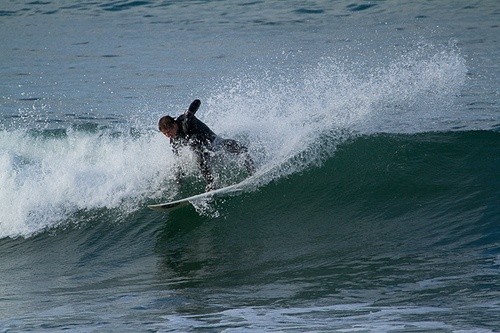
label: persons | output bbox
[158,99,256,192]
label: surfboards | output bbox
[146,167,273,215]
